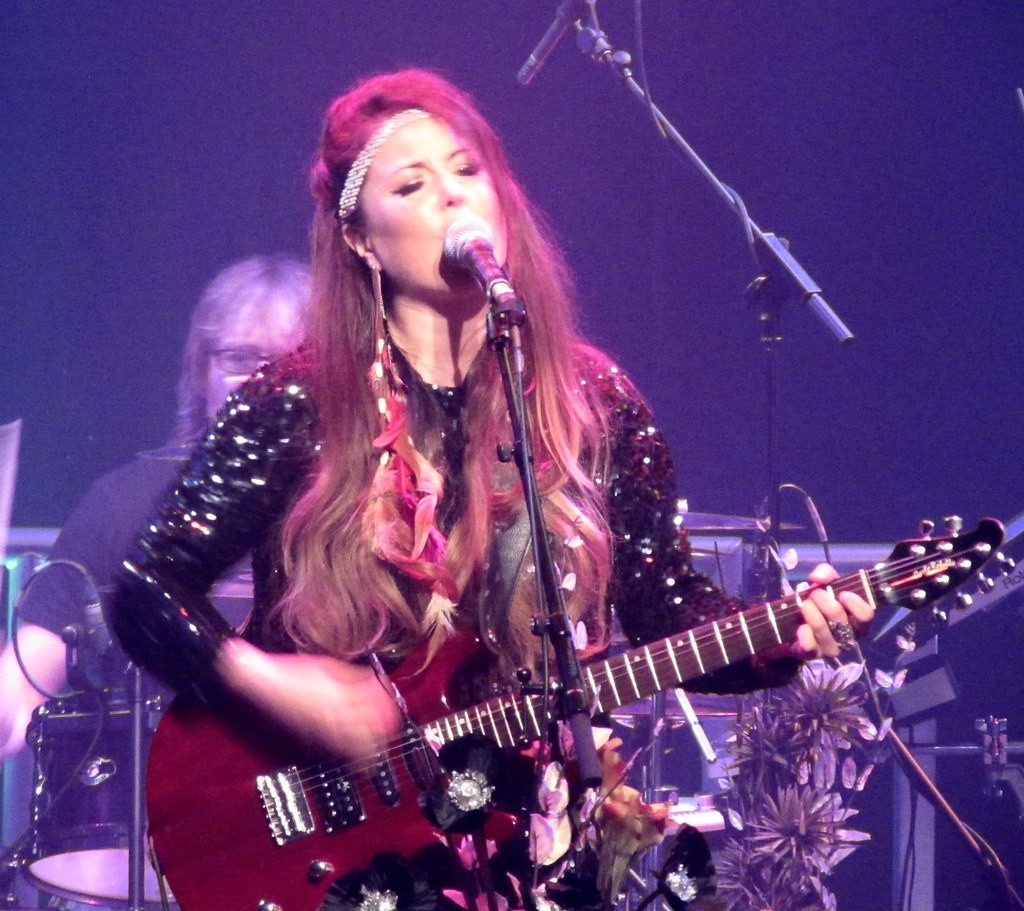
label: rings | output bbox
[832,620,854,645]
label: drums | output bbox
[19,688,183,911]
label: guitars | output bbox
[144,512,1007,910]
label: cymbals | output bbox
[609,698,749,716]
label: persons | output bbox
[0,252,315,759]
[105,65,874,910]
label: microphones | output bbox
[79,574,114,687]
[743,495,770,600]
[515,0,583,85]
[444,215,522,325]
